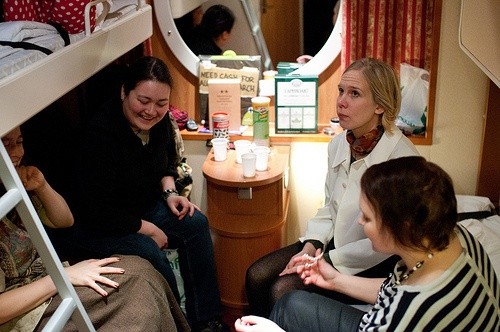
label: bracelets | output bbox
[163,189,179,197]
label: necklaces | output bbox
[390,251,437,287]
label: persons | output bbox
[186,4,236,55]
[245,58,423,332]
[76,56,223,332]
[0,124,192,332]
[235,156,500,332]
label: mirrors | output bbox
[153,0,344,96]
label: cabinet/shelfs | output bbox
[202,143,290,332]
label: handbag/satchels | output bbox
[393,63,431,134]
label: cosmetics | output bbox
[322,116,340,135]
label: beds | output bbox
[0,6,153,140]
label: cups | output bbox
[211,138,229,161]
[241,154,256,177]
[250,146,270,171]
[234,140,251,164]
[211,111,230,151]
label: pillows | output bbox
[1,0,95,34]
